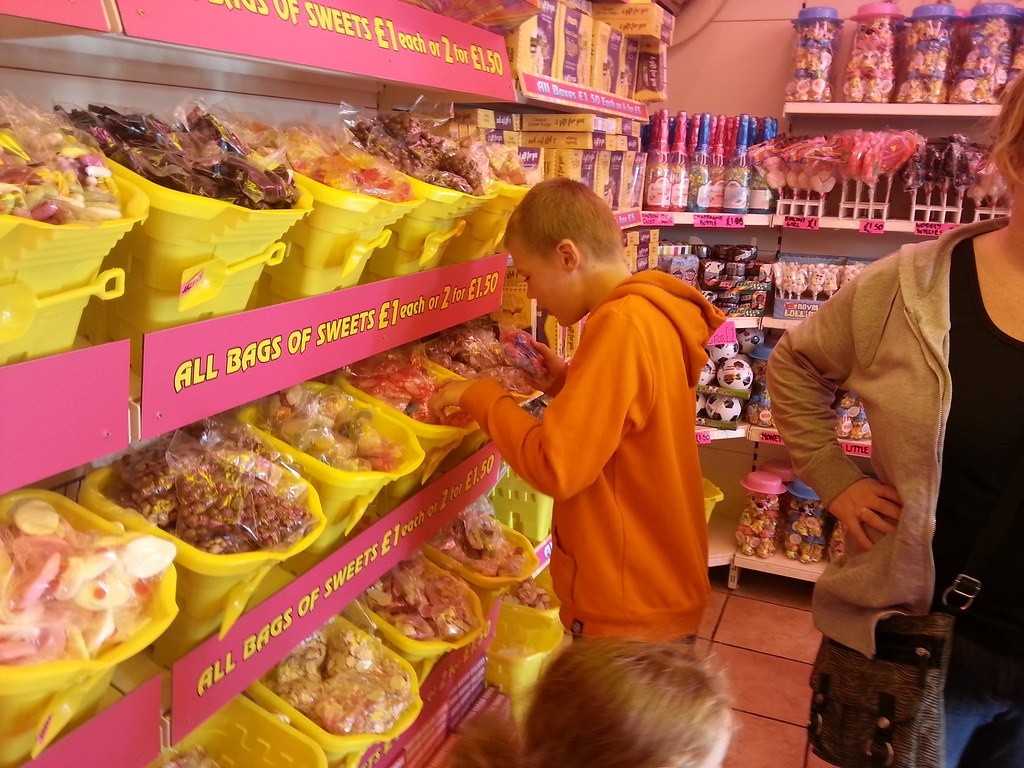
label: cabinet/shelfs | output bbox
[0,0,552,768]
[514,1,666,579]
[727,101,1007,590]
[506,67,650,268]
[0,0,512,768]
[639,209,774,568]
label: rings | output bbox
[857,507,868,517]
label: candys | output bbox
[734,14,1024,557]
[1,78,549,735]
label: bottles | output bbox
[734,346,872,563]
[785,3,1024,104]
[640,108,776,214]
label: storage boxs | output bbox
[0,0,726,768]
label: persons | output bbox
[443,636,734,768]
[766,73,1024,768]
[424,177,727,642]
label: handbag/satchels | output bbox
[808,610,955,768]
